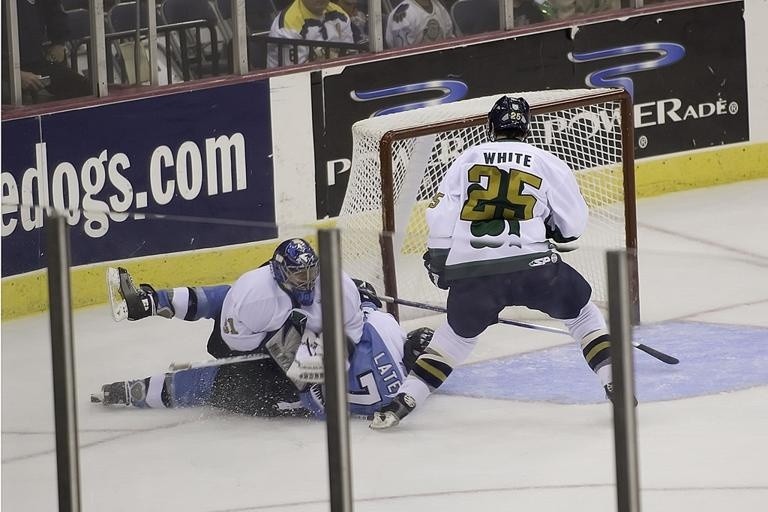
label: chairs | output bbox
[46,0,279,84]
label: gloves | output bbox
[423,247,454,290]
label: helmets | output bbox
[272,237,319,305]
[402,326,435,375]
[488,95,532,142]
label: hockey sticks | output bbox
[375,294,680,365]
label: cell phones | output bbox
[38,76,52,87]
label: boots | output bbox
[606,384,638,408]
[375,392,416,422]
[101,381,132,405]
[118,266,157,322]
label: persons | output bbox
[1,0,59,106]
[102,266,435,419]
[368,96,640,420]
[207,239,365,382]
[265,0,456,72]
